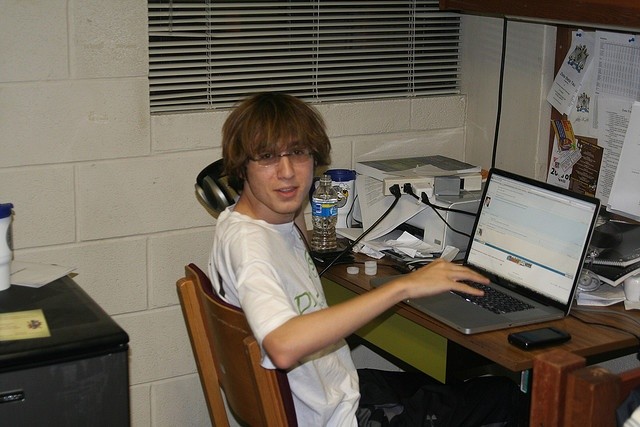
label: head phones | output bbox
[195,157,244,213]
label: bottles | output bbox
[310,174,338,252]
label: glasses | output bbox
[248,147,315,167]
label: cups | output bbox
[323,168,356,238]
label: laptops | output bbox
[369,167,602,335]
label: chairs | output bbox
[175,263,302,426]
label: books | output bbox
[355,153,482,181]
[583,261,640,288]
[335,227,405,251]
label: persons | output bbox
[484,196,490,207]
[477,227,482,235]
[208,90,528,427]
[555,161,560,168]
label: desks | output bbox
[307,226,639,385]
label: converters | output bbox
[434,176,461,196]
[412,182,434,198]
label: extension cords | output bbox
[382,173,483,196]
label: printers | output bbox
[352,175,487,262]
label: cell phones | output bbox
[507,327,572,351]
[312,247,346,254]
